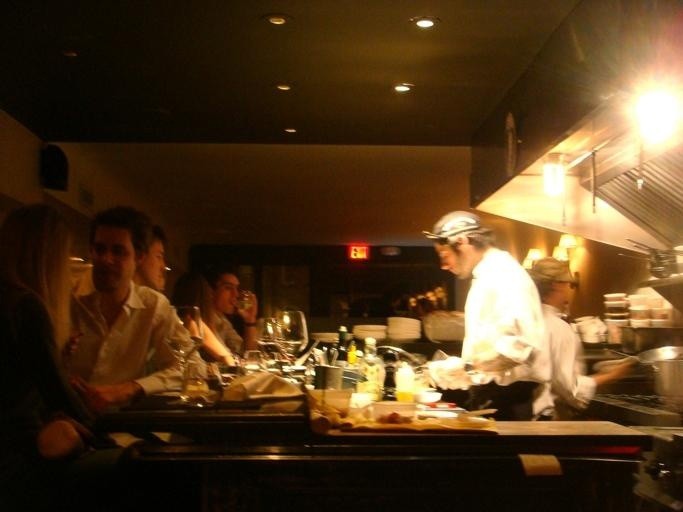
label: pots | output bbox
[603,346,682,399]
[616,237,683,278]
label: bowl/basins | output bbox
[577,291,670,344]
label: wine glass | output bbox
[163,303,202,405]
[255,310,307,362]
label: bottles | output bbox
[336,325,359,365]
[354,336,384,400]
[394,360,415,402]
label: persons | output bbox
[0,200,98,509]
[132,223,168,289]
[56,204,210,415]
[527,255,630,421]
[418,209,556,421]
[185,264,259,366]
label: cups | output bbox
[231,291,252,311]
[313,364,343,390]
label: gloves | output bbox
[422,356,471,391]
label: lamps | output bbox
[541,153,568,199]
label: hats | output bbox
[526,257,575,285]
[432,209,494,241]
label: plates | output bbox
[310,331,352,343]
[353,322,387,340]
[385,316,421,343]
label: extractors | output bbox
[579,118,682,248]
